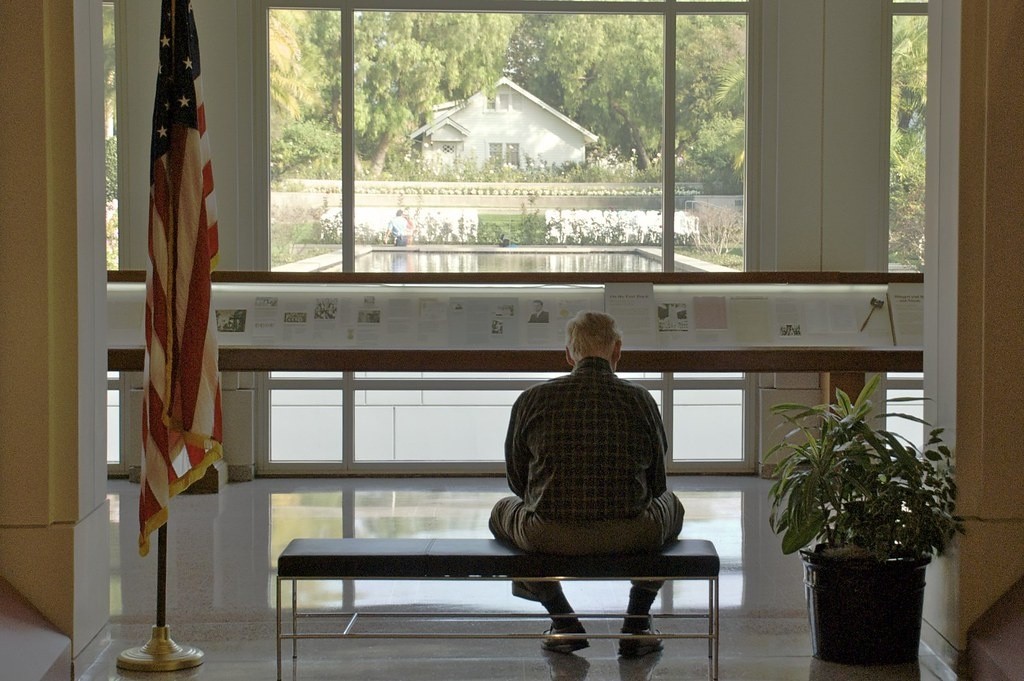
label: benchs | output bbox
[275,538,722,681]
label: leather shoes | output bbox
[541,628,590,653]
[619,629,664,657]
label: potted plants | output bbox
[761,371,968,666]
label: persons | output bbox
[489,309,685,656]
[528,300,549,323]
[385,210,413,246]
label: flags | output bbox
[138,0,224,556]
